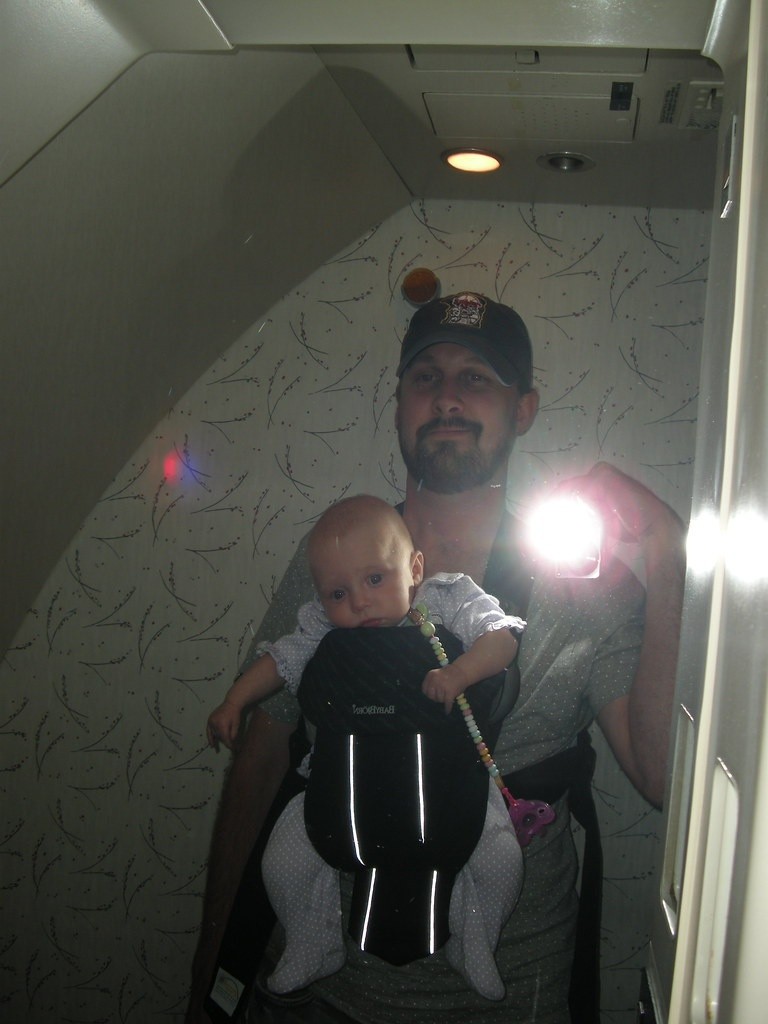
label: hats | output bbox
[396,292,532,387]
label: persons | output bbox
[206,490,528,1001]
[181,291,687,1024]
